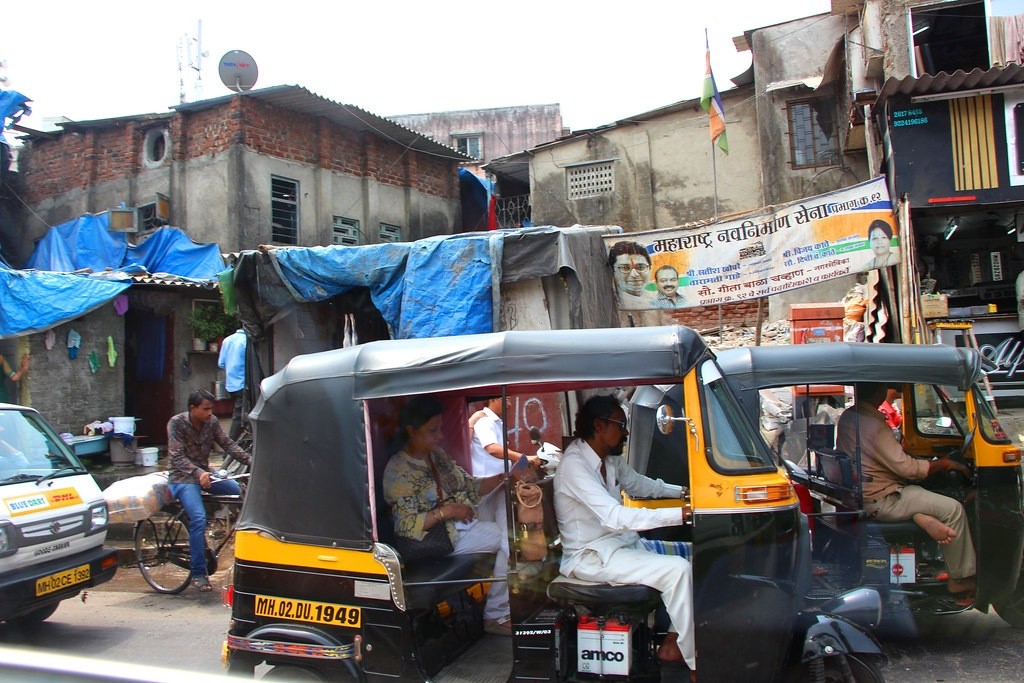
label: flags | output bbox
[700,37,730,157]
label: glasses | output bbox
[614,265,649,274]
[599,416,627,430]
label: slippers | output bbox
[955,591,975,606]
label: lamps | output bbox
[944,218,960,240]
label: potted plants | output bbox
[185,305,216,351]
[198,316,224,352]
[213,306,240,352]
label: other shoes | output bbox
[484,616,511,636]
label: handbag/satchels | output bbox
[393,521,454,562]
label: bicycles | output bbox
[131,471,250,596]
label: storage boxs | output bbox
[921,295,949,318]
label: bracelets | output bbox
[469,426,474,429]
[432,509,441,522]
[686,506,692,521]
[681,486,688,500]
[438,507,446,521]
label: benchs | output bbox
[373,455,496,609]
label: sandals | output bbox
[191,576,213,591]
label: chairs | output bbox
[818,447,920,539]
[514,478,659,614]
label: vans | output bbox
[0,402,118,630]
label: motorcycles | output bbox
[619,343,1024,633]
[223,323,890,683]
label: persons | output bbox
[554,394,697,683]
[835,381,978,593]
[1015,271,1024,345]
[609,240,688,309]
[860,220,901,270]
[166,389,253,592]
[0,353,29,404]
[469,394,541,479]
[383,397,518,637]
[218,320,248,460]
